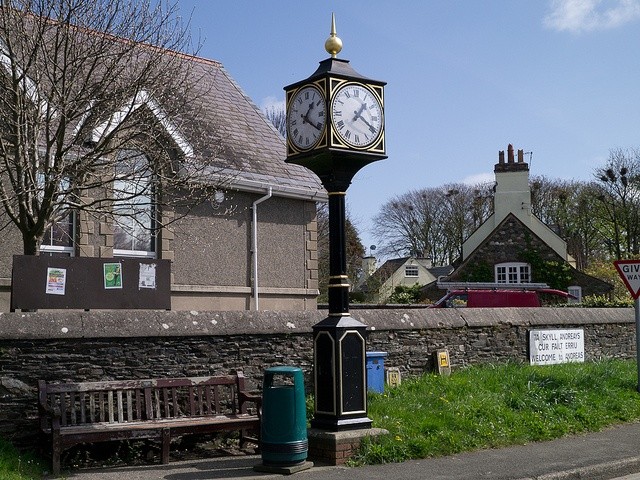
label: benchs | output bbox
[39,370,262,474]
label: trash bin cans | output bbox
[259,365,308,466]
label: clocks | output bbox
[328,77,386,154]
[286,83,328,152]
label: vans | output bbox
[427,289,577,308]
